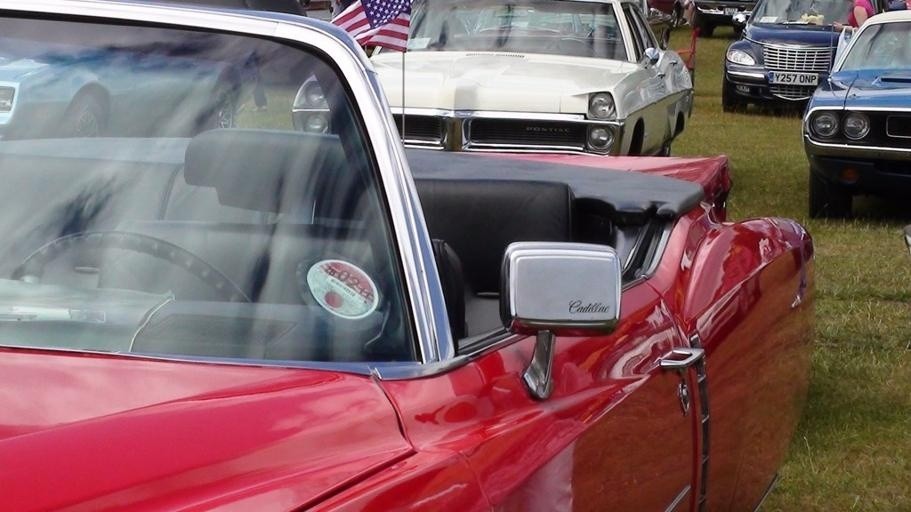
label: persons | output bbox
[329,0,343,20]
[848,0,876,30]
[886,0,907,12]
[682,0,696,26]
[214,33,268,115]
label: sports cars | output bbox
[0,0,815,512]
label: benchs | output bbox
[3,154,577,349]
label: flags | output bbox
[328,0,415,53]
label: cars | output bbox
[802,9,910,213]
[693,0,760,38]
[0,37,249,138]
[290,0,695,166]
[722,0,880,112]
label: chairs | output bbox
[103,123,457,356]
